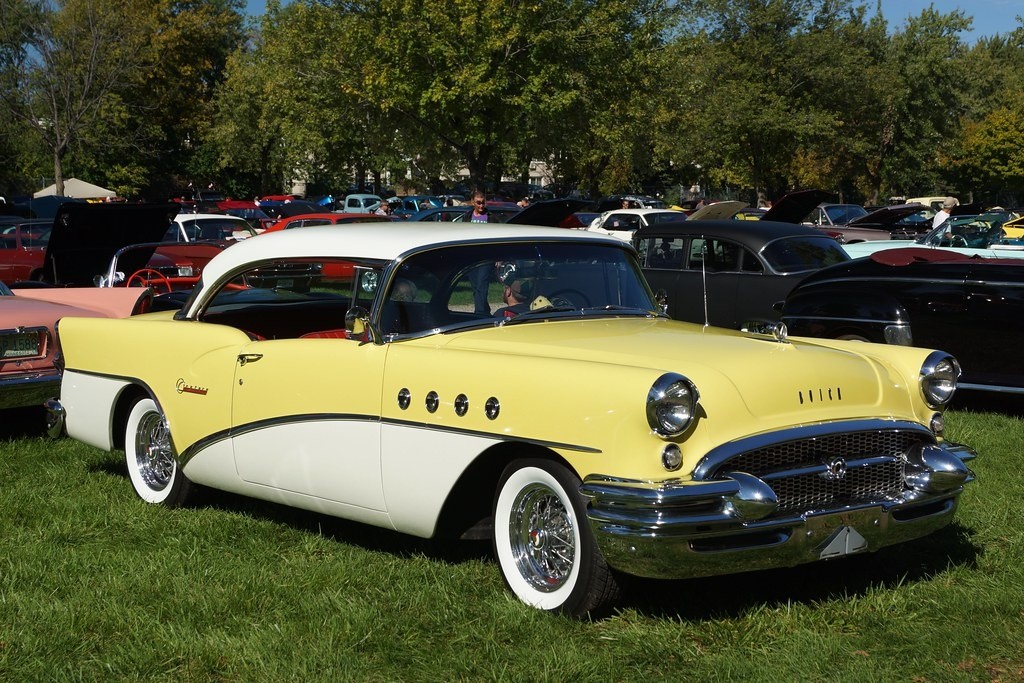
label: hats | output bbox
[504,271,530,295]
[943,197,958,208]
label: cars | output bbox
[1,190,1024,416]
[56,218,981,627]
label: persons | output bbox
[493,271,537,317]
[441,198,453,221]
[932,196,955,239]
[695,198,707,209]
[622,201,629,209]
[462,192,501,315]
[374,201,389,216]
[517,198,529,207]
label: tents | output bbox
[34,178,116,198]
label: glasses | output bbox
[476,200,486,204]
[382,204,389,207]
